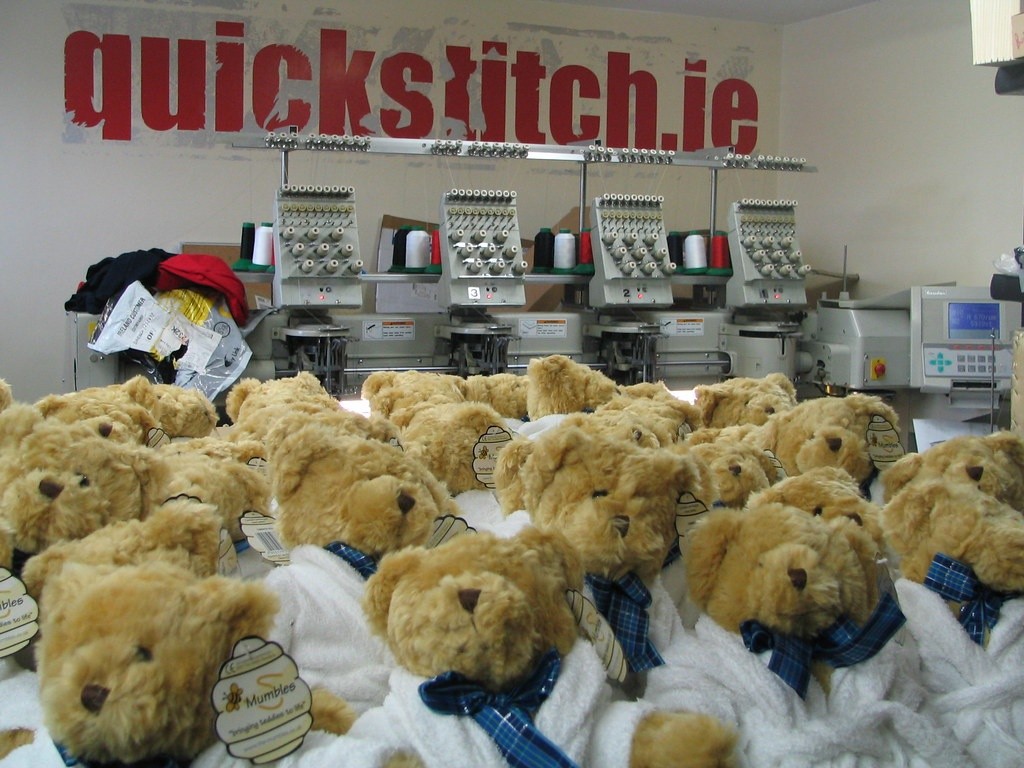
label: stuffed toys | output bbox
[0,356,1024,768]
[0,562,422,768]
